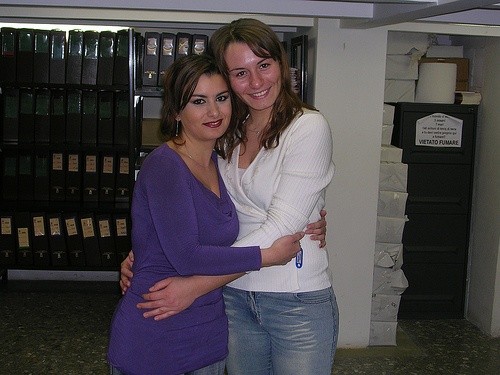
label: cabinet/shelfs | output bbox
[395,101,476,319]
[1,16,216,295]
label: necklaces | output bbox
[250,113,271,136]
[184,146,211,168]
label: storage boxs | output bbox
[368,31,471,346]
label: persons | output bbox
[120,18,339,375]
[107,54,328,375]
